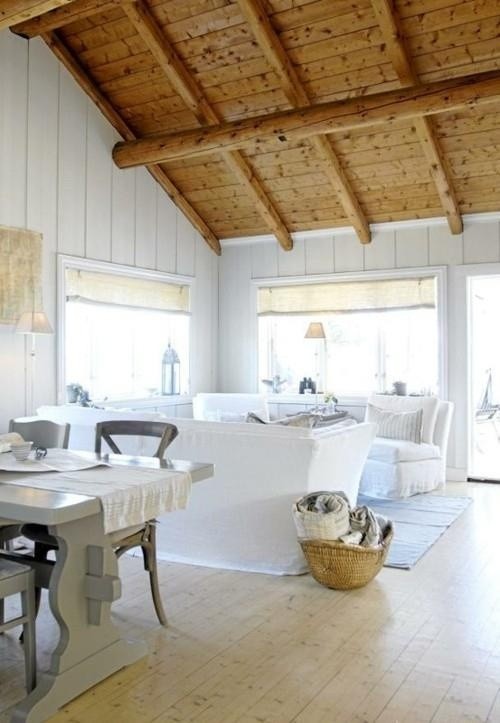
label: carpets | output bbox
[356,493,472,569]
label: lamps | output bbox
[305,322,325,338]
[15,313,53,355]
[161,344,180,395]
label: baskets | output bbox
[296,521,394,591]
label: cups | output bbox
[11,442,32,462]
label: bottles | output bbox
[67,385,77,403]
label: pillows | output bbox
[368,403,422,445]
[245,412,320,428]
[36,406,165,420]
[365,395,440,446]
[197,393,269,419]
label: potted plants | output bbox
[67,384,92,403]
[324,393,338,414]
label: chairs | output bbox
[359,400,454,500]
[0,558,39,693]
[9,421,71,451]
[192,396,270,421]
[18,422,177,645]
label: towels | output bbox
[0,464,192,535]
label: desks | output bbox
[286,414,354,427]
[0,448,213,723]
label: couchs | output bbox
[11,418,380,576]
